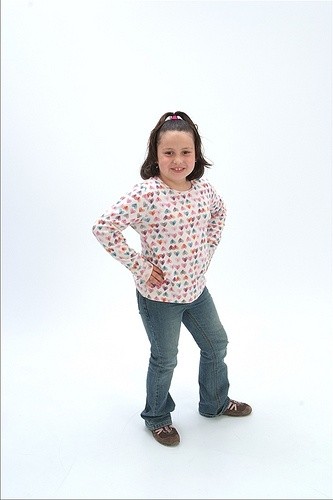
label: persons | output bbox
[92,111,252,448]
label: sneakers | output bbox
[223,399,251,417]
[151,424,180,447]
